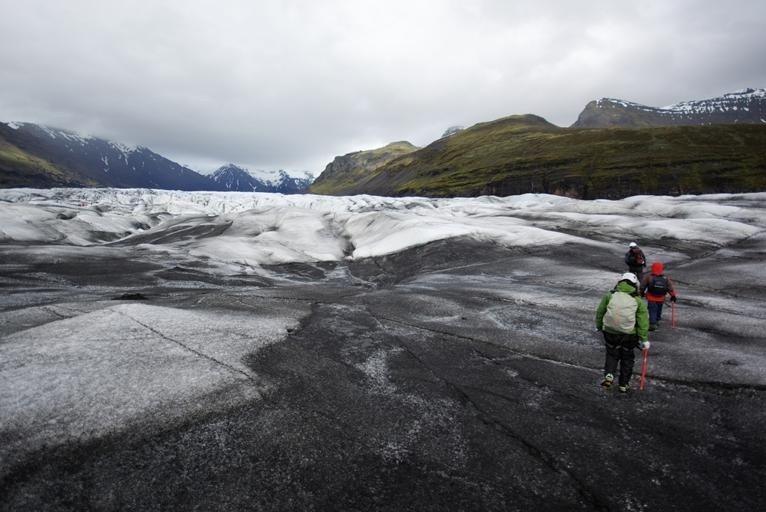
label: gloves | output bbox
[670,296,677,303]
[641,341,650,350]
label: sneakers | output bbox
[600,373,614,390]
[648,323,656,331]
[618,384,631,393]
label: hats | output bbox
[629,242,637,247]
[622,272,638,284]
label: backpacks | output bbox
[602,289,640,334]
[648,274,667,296]
[630,250,644,266]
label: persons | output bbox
[625,242,676,330]
[596,272,650,392]
